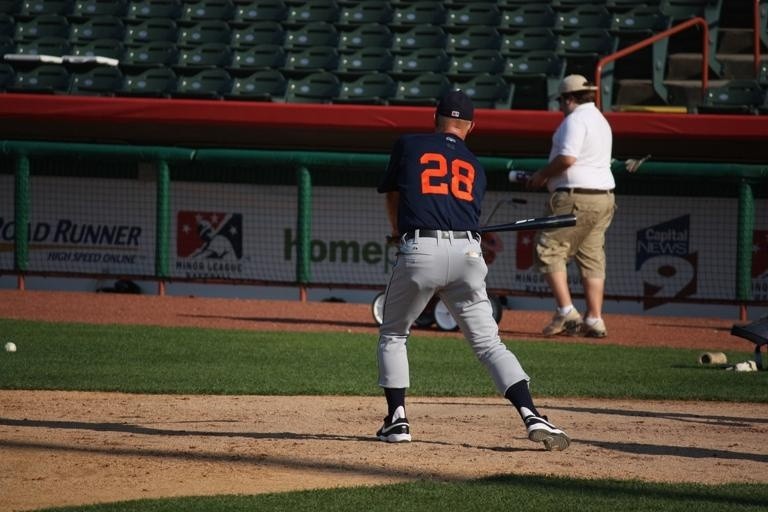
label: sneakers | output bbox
[561,316,610,338]
[521,414,572,452]
[540,306,585,337]
[375,415,414,444]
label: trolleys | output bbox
[366,198,531,331]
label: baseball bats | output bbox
[194,213,234,255]
[385,214,576,244]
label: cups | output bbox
[698,351,728,365]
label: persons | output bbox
[376,95,572,450]
[190,220,237,262]
[532,74,615,338]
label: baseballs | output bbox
[5,341,17,352]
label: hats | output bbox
[547,73,600,102]
[436,88,476,122]
